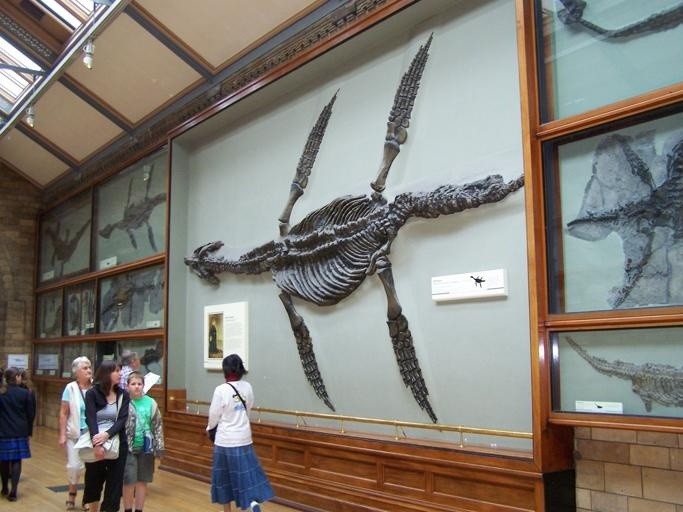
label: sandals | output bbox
[65,492,76,509]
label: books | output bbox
[141,372,161,394]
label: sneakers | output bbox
[250,501,262,512]
[1,487,17,501]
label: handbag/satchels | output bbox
[208,424,219,443]
[73,429,120,463]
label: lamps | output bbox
[23,105,39,127]
[83,37,96,70]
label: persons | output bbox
[122,372,162,512]
[118,350,140,392]
[80,360,128,512]
[7,368,35,478]
[205,354,276,511]
[0,365,35,501]
[58,355,89,512]
